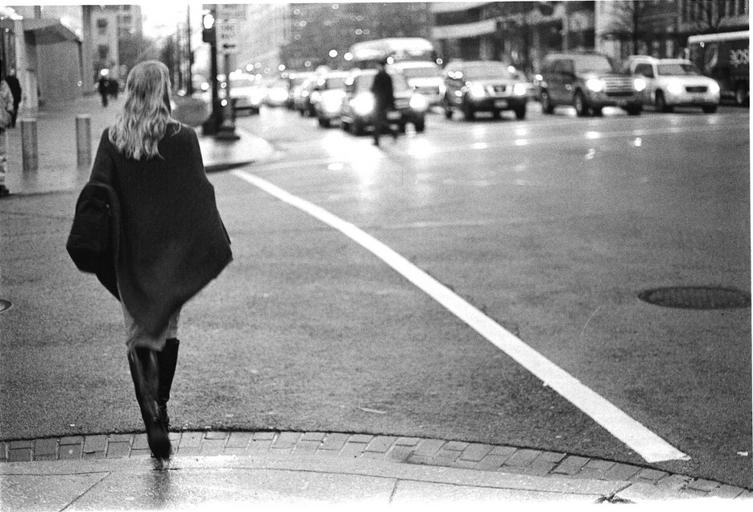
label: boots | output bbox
[152,336,181,438]
[123,342,173,465]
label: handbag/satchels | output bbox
[63,195,118,277]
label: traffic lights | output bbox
[202,13,215,42]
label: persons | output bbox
[70,58,212,462]
[369,58,398,146]
[0,71,13,196]
[96,72,118,109]
